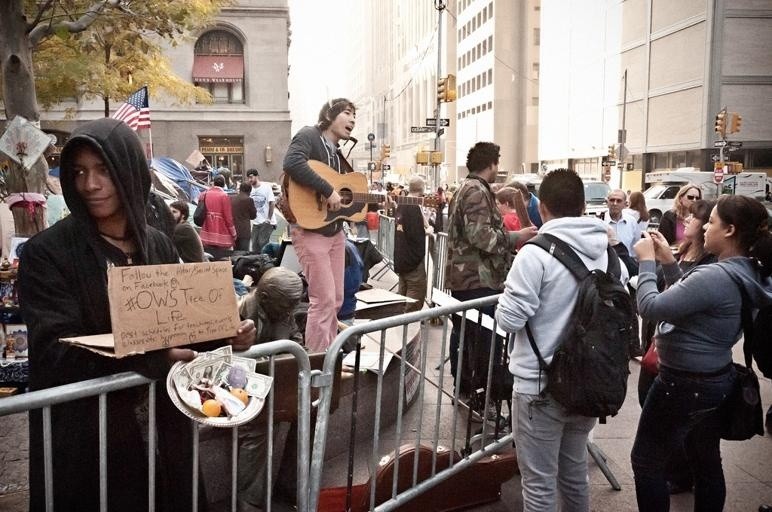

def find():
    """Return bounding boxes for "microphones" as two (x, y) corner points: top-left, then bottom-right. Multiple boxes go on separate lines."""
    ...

(349, 136), (359, 144)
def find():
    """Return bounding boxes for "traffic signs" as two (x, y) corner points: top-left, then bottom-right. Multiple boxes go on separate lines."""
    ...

(438, 128), (444, 137)
(425, 117), (450, 128)
(601, 160), (616, 166)
(713, 139), (743, 152)
(710, 153), (730, 162)
(411, 126), (435, 132)
(383, 164), (391, 170)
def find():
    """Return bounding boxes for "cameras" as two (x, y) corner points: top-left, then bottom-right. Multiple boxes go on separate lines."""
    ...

(641, 232), (653, 238)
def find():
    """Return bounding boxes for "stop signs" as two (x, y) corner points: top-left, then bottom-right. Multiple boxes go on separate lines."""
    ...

(713, 167), (723, 184)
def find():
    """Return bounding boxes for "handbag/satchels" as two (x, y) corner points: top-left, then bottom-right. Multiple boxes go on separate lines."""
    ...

(193, 191), (210, 228)
(718, 360), (765, 443)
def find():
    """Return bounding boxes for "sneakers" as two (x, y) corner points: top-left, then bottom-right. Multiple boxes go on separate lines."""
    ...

(469, 403), (512, 423)
(448, 390), (480, 407)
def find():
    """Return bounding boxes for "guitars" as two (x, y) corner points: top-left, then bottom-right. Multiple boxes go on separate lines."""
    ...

(280, 159), (441, 230)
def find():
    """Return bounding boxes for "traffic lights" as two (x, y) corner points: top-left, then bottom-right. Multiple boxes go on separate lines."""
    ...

(437, 77), (450, 102)
(713, 111), (726, 134)
(608, 144), (615, 158)
(731, 112), (742, 134)
(382, 143), (391, 158)
(368, 162), (376, 171)
(626, 162), (634, 171)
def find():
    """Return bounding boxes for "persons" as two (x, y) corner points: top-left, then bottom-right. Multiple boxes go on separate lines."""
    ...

(20, 100), (771, 512)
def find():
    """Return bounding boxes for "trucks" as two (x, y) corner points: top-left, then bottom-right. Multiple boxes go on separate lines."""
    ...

(640, 169), (768, 230)
(583, 177), (616, 222)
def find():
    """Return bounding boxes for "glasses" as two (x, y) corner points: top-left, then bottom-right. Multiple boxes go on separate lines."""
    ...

(684, 194), (700, 201)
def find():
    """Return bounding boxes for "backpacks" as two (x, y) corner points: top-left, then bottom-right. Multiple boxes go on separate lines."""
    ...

(518, 234), (633, 425)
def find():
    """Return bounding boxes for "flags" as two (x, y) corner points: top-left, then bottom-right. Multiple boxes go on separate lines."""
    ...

(113, 85), (152, 128)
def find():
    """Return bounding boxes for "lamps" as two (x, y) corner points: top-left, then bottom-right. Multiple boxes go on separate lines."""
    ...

(265, 144), (272, 163)
